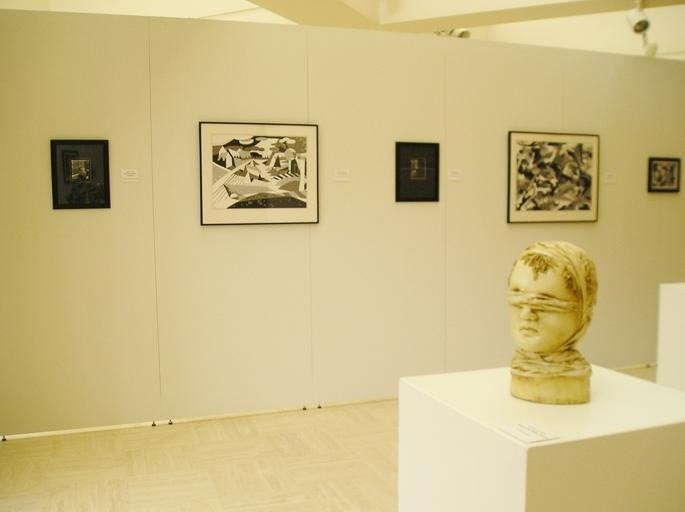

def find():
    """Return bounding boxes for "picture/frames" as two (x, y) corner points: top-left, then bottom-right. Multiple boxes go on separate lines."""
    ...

(395, 142), (439, 202)
(199, 121), (319, 225)
(507, 130), (599, 223)
(648, 157), (680, 192)
(50, 139), (111, 209)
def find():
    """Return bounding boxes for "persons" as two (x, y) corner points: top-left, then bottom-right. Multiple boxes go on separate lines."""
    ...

(505, 237), (600, 378)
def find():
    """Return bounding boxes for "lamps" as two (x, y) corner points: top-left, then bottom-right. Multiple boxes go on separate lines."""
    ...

(626, 8), (659, 60)
(433, 26), (471, 39)
